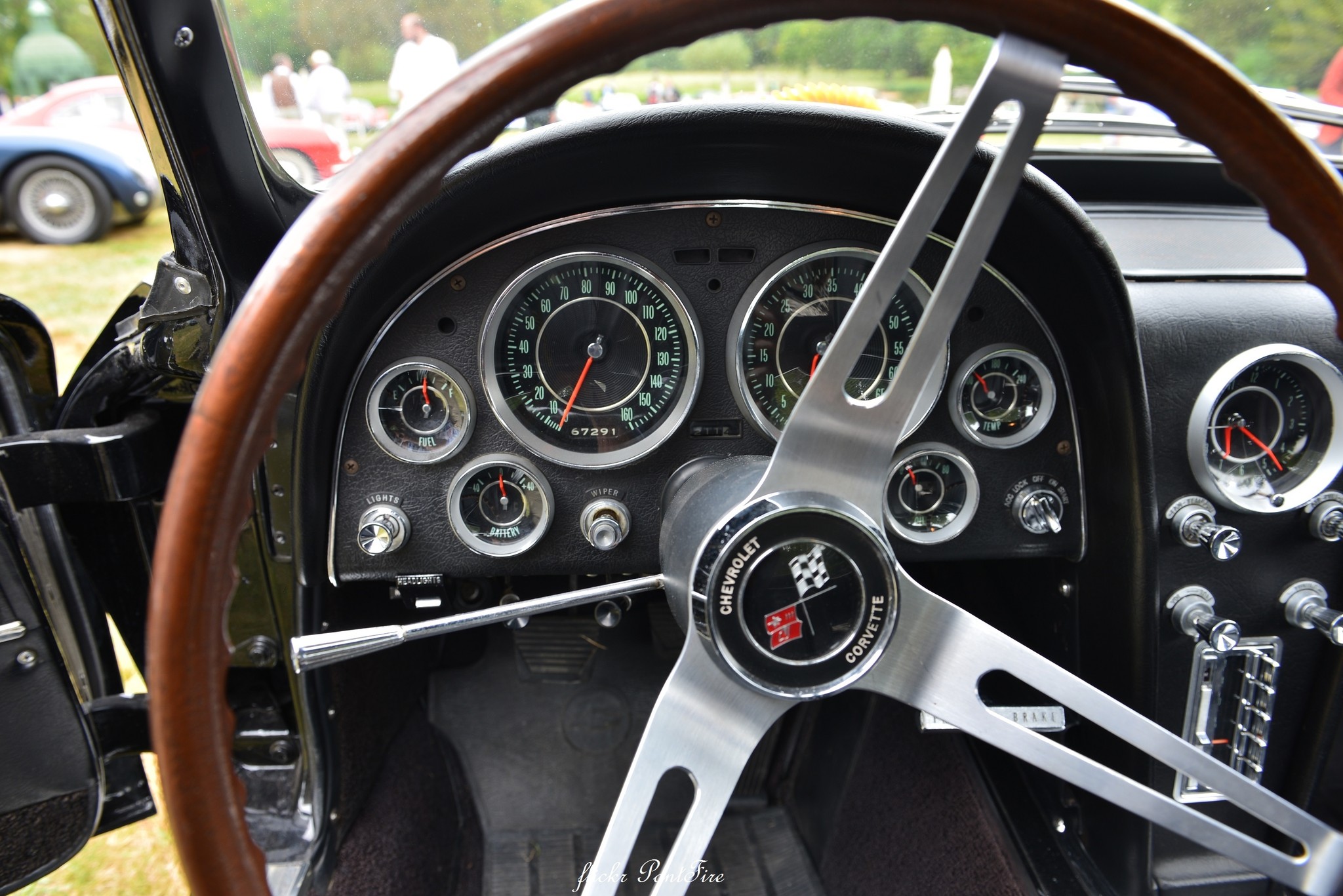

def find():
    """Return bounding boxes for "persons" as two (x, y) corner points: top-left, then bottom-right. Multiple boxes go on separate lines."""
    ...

(301, 50), (351, 127)
(386, 14), (459, 107)
(260, 52), (305, 122)
(526, 86), (680, 130)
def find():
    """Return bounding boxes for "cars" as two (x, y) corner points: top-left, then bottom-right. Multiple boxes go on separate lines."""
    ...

(0, 75), (343, 247)
